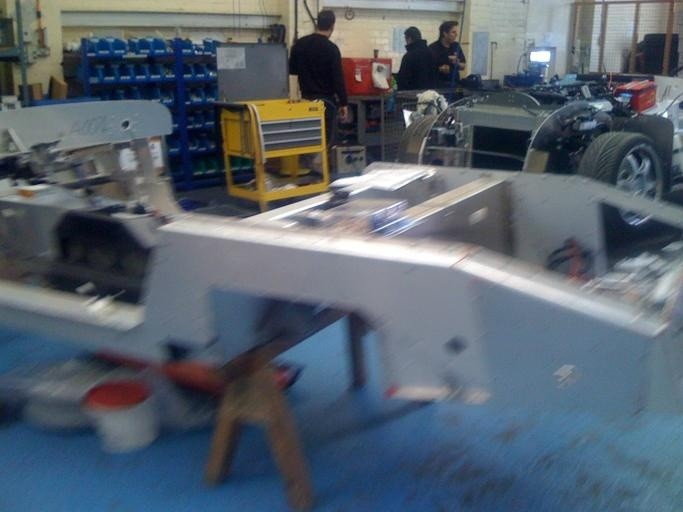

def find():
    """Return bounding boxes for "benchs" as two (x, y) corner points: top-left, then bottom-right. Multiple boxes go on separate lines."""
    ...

(205, 306), (365, 512)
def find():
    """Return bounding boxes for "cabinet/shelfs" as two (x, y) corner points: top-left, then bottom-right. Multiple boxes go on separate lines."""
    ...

(80, 37), (255, 191)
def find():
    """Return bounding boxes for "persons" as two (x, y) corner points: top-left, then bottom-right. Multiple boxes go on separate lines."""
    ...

(430, 21), (467, 88)
(395, 26), (432, 90)
(288, 10), (348, 144)
